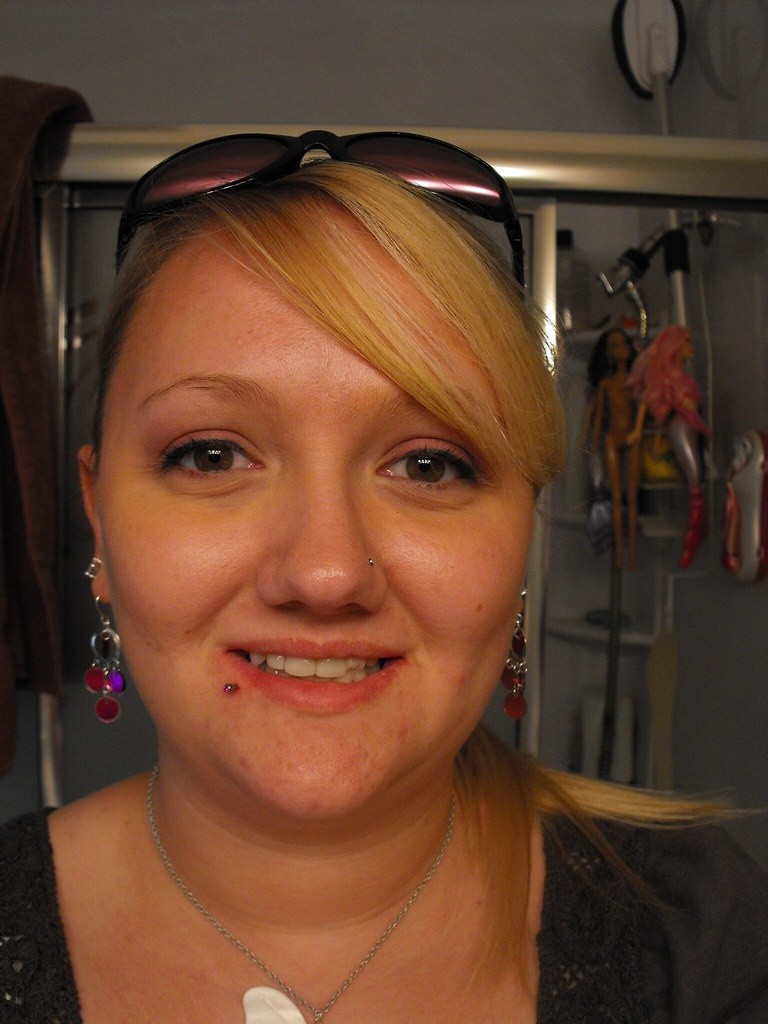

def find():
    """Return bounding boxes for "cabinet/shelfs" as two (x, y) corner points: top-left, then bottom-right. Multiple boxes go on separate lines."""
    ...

(527, 331), (672, 790)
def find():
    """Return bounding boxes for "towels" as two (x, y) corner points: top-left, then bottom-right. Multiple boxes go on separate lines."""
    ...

(0, 75), (91, 742)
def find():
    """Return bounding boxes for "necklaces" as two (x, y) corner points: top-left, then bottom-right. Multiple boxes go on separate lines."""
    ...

(145, 768), (457, 1023)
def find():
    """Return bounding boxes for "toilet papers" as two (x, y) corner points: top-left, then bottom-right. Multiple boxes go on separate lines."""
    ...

(579, 693), (635, 784)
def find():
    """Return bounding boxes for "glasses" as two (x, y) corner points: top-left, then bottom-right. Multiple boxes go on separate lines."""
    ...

(114, 130), (524, 302)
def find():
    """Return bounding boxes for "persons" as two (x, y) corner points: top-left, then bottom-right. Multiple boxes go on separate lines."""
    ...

(0, 160), (768, 1024)
